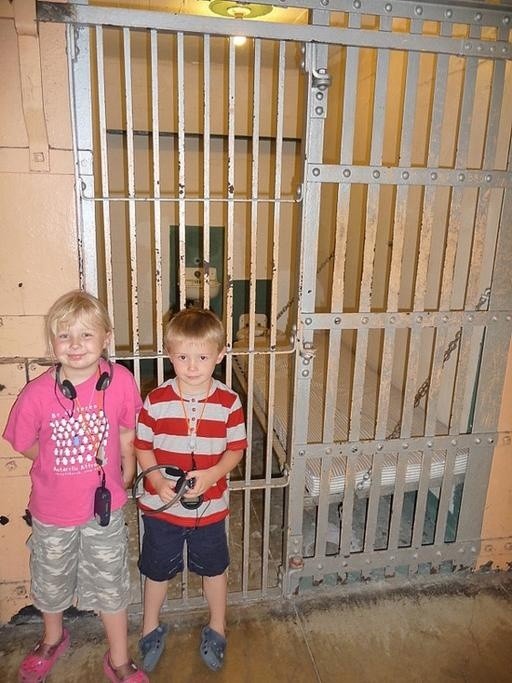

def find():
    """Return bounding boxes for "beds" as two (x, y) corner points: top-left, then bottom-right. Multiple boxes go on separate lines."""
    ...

(230, 327), (469, 550)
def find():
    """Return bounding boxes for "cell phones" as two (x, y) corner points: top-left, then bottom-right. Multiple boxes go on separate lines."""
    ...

(93, 486), (110, 527)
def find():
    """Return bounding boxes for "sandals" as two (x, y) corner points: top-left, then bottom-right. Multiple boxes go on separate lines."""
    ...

(102, 647), (149, 683)
(139, 622), (169, 671)
(19, 629), (70, 683)
(201, 626), (227, 671)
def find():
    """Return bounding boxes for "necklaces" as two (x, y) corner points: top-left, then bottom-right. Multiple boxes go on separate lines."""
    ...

(177, 376), (213, 434)
(60, 361), (103, 451)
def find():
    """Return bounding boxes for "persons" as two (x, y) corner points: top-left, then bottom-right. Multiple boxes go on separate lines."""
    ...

(1, 286), (150, 683)
(132, 306), (249, 676)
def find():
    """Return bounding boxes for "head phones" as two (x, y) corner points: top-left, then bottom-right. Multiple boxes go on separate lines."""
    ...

(132, 464), (204, 514)
(56, 353), (113, 400)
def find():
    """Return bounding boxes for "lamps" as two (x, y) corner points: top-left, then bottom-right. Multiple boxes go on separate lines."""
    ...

(226, 6), (251, 47)
